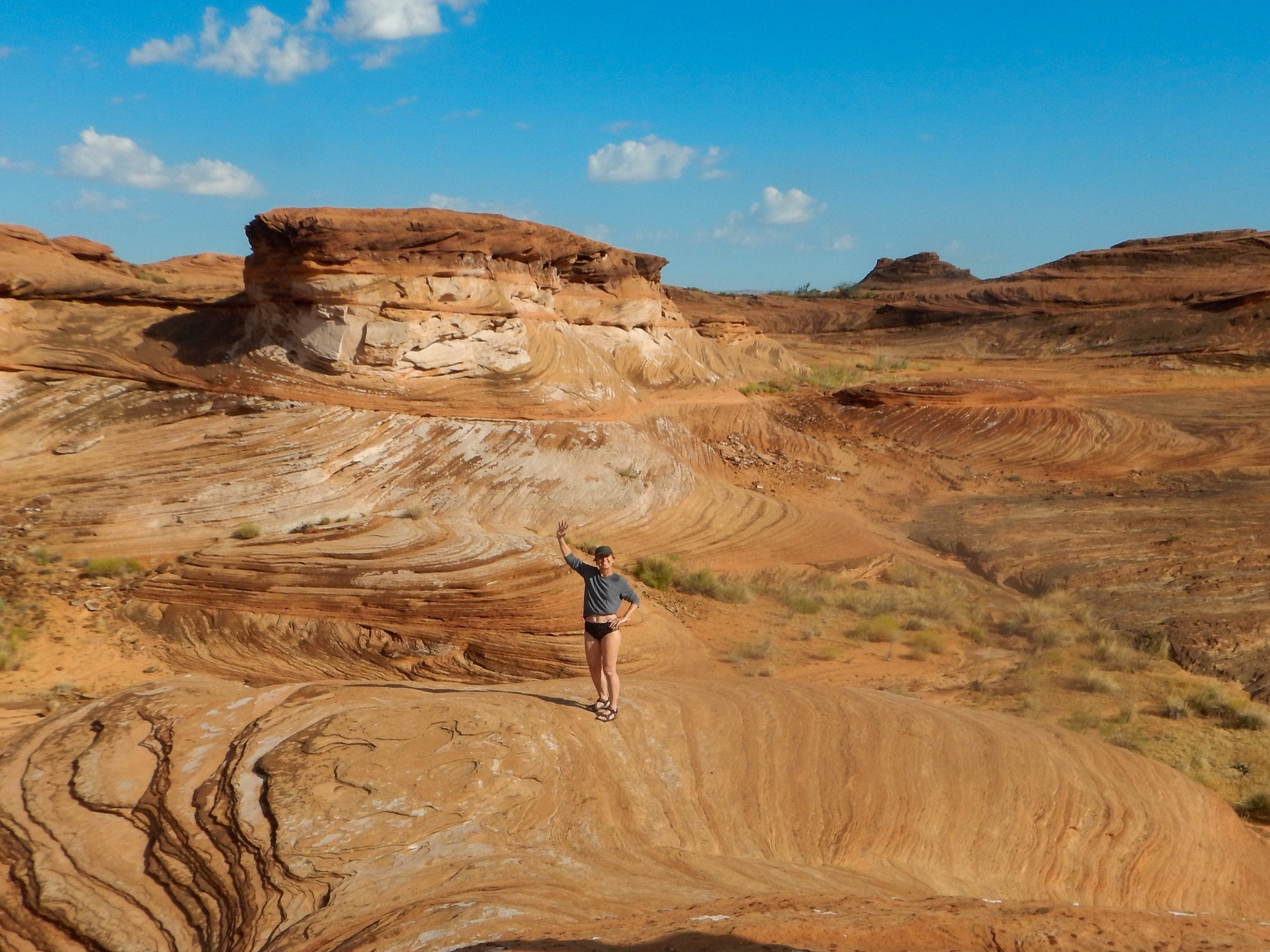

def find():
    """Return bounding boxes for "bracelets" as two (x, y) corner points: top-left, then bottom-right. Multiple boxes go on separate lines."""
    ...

(622, 615), (628, 622)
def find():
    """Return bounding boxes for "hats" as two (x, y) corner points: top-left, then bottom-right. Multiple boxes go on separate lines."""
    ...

(594, 545), (613, 556)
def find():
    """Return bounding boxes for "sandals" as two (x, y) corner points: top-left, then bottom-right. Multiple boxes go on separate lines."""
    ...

(586, 697), (610, 712)
(596, 707), (618, 721)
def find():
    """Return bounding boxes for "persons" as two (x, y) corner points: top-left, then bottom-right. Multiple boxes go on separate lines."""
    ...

(557, 520), (640, 722)
